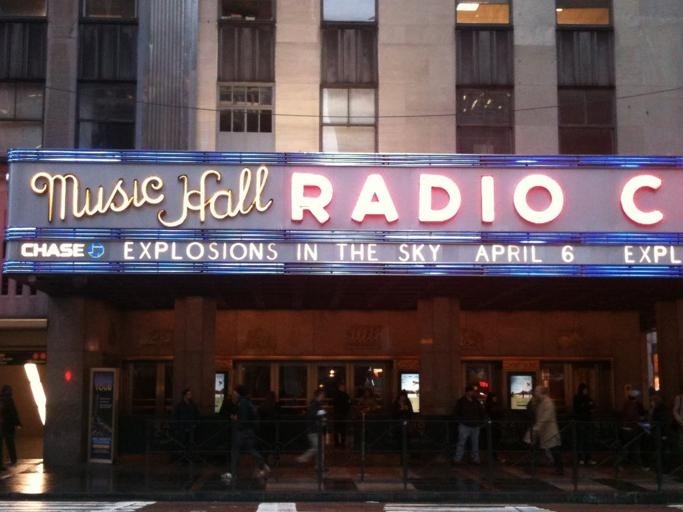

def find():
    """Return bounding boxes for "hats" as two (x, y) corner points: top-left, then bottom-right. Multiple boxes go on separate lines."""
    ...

(465, 385), (473, 392)
(233, 383), (250, 396)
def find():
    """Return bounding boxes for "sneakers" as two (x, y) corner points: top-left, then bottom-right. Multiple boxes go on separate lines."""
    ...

(223, 469), (236, 481)
(253, 468), (267, 479)
(579, 458), (598, 467)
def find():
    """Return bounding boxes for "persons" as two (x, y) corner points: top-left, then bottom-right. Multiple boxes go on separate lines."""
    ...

(387, 388), (418, 465)
(453, 386), (486, 468)
(172, 388), (199, 465)
(619, 387), (682, 484)
(293, 389), (329, 471)
(0, 383), (24, 468)
(357, 387), (376, 405)
(218, 385), (270, 482)
(527, 384), (560, 463)
(326, 379), (353, 451)
(571, 382), (598, 464)
(257, 391), (281, 465)
